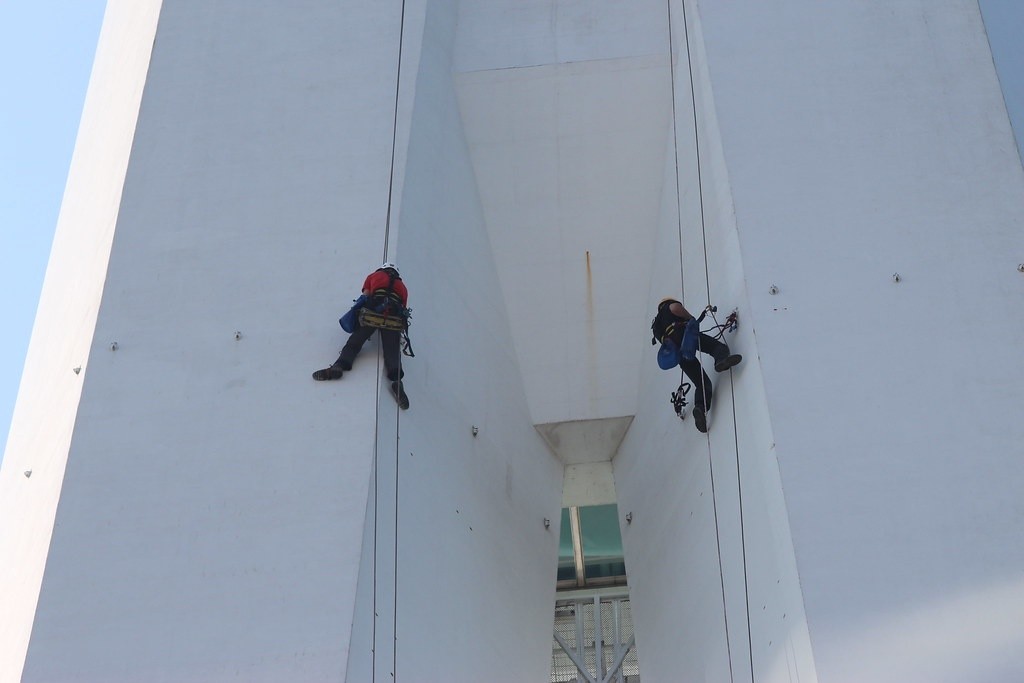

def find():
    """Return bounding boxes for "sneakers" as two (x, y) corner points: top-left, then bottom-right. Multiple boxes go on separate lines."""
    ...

(715, 354), (742, 372)
(693, 406), (707, 433)
(392, 381), (409, 410)
(312, 367), (342, 381)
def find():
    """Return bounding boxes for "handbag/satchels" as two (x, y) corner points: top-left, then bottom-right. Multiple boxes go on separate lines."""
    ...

(339, 300), (366, 333)
(657, 337), (680, 370)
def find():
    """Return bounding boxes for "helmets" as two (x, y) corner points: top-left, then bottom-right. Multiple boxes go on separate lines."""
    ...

(381, 262), (400, 275)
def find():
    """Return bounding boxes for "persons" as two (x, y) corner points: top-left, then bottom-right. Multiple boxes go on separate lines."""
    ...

(650, 297), (743, 433)
(311, 262), (410, 410)
